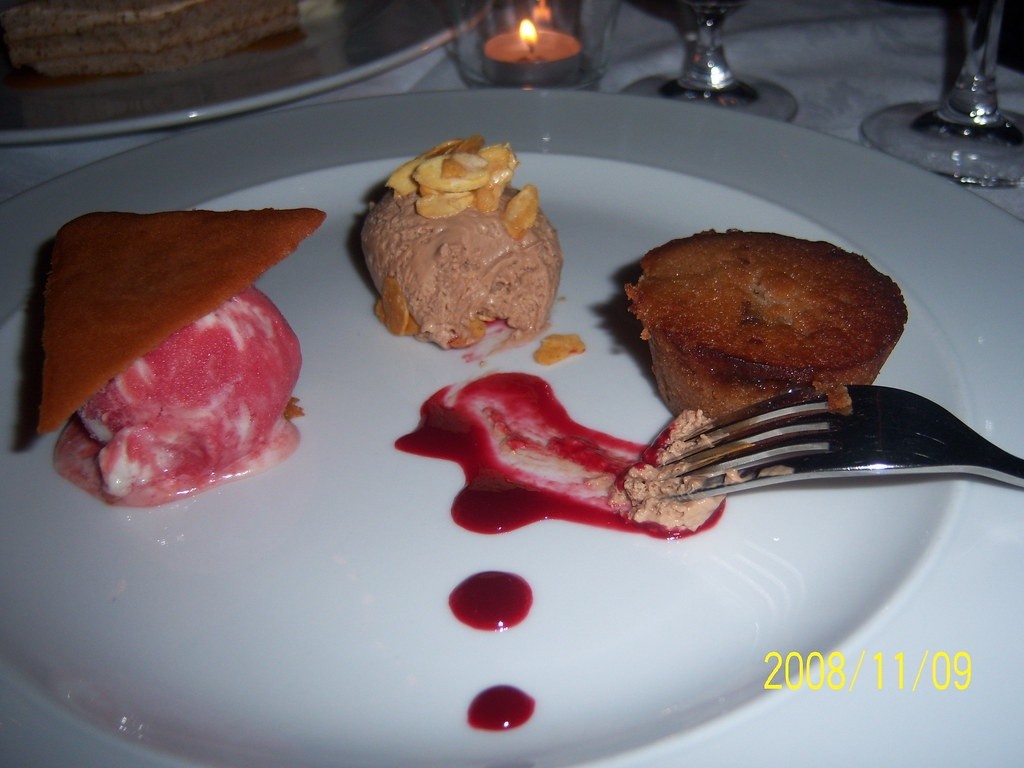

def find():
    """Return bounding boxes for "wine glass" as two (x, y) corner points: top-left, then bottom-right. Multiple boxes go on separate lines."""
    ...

(616, 0), (798, 124)
(858, 1), (1024, 188)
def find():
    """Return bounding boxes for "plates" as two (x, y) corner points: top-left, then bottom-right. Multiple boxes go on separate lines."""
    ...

(0, 0), (492, 145)
(0, 154), (966, 767)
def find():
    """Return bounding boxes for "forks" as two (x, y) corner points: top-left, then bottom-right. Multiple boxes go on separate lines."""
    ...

(663, 385), (1024, 505)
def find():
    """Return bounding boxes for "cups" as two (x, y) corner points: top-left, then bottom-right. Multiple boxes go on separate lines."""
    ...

(451, 0), (622, 90)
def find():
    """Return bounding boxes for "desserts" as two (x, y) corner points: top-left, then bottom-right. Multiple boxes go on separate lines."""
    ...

(38, 206), (327, 500)
(360, 134), (560, 351)
(615, 414), (731, 531)
